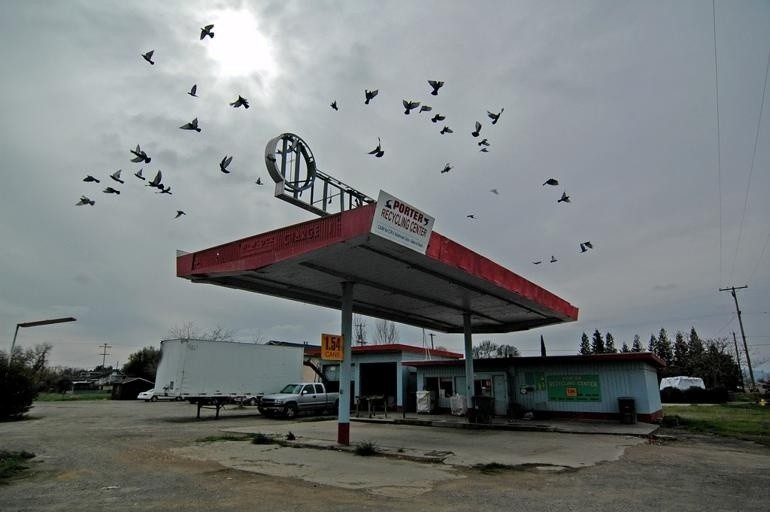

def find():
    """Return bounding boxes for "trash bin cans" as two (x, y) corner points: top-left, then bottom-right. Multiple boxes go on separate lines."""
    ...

(617, 396), (635, 425)
(471, 393), (494, 422)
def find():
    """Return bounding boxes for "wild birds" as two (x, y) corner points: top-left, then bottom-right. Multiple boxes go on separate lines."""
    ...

(400, 81), (506, 153)
(330, 89), (379, 111)
(141, 50), (154, 65)
(490, 188), (499, 195)
(543, 178), (572, 204)
(230, 95), (250, 109)
(75, 142), (186, 219)
(466, 214), (475, 219)
(580, 241), (594, 254)
(368, 137), (384, 158)
(532, 255), (558, 265)
(218, 155), (233, 174)
(179, 84), (201, 133)
(199, 24), (215, 40)
(256, 177), (263, 186)
(439, 164), (451, 174)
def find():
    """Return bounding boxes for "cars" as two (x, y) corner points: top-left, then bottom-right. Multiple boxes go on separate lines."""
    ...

(136, 388), (185, 402)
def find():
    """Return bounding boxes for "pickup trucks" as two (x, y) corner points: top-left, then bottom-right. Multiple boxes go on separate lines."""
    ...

(257, 382), (339, 421)
